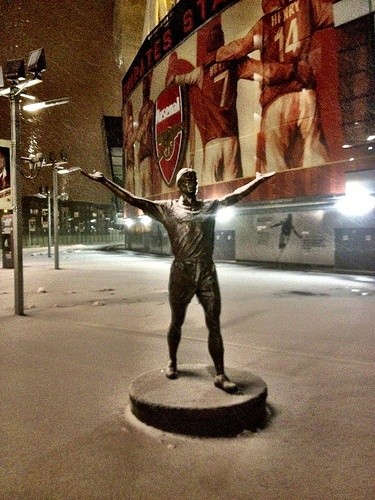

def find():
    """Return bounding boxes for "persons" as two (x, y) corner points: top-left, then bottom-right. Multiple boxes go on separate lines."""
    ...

(167, 23), (313, 187)
(77, 167), (278, 394)
(123, 100), (154, 195)
(201, 0), (337, 173)
(135, 74), (155, 195)
(165, 51), (196, 169)
(271, 213), (302, 250)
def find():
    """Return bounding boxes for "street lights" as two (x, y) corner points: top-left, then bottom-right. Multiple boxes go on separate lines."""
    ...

(0, 48), (71, 316)
(20, 134), (80, 269)
(34, 185), (69, 258)
